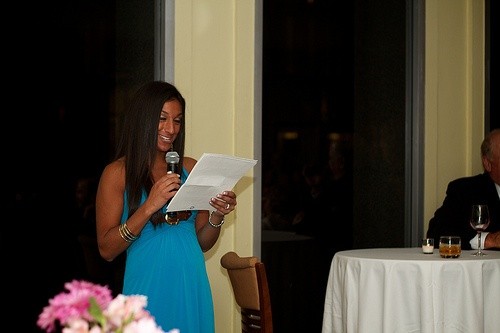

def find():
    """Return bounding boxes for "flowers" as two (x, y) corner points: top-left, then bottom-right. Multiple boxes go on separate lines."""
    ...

(36, 281), (181, 333)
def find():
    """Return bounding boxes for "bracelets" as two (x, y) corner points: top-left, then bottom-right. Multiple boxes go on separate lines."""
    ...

(209, 214), (224, 227)
(119, 222), (136, 242)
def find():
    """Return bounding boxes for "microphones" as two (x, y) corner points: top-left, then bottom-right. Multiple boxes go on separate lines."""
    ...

(165, 152), (180, 225)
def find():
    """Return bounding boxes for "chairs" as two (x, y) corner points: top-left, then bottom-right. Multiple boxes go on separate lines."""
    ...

(220, 252), (273, 333)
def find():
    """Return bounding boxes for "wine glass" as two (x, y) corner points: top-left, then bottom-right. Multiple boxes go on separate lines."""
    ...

(470, 205), (490, 256)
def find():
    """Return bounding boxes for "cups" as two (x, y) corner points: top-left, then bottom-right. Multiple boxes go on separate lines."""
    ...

(422, 238), (434, 254)
(438, 236), (461, 258)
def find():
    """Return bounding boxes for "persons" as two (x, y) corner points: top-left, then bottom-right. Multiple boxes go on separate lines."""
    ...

(426, 125), (500, 249)
(97, 81), (236, 333)
(264, 141), (354, 249)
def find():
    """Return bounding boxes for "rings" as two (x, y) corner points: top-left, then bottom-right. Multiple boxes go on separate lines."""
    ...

(225, 204), (229, 209)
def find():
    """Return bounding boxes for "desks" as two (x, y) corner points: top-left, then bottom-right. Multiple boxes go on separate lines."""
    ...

(321, 248), (500, 333)
